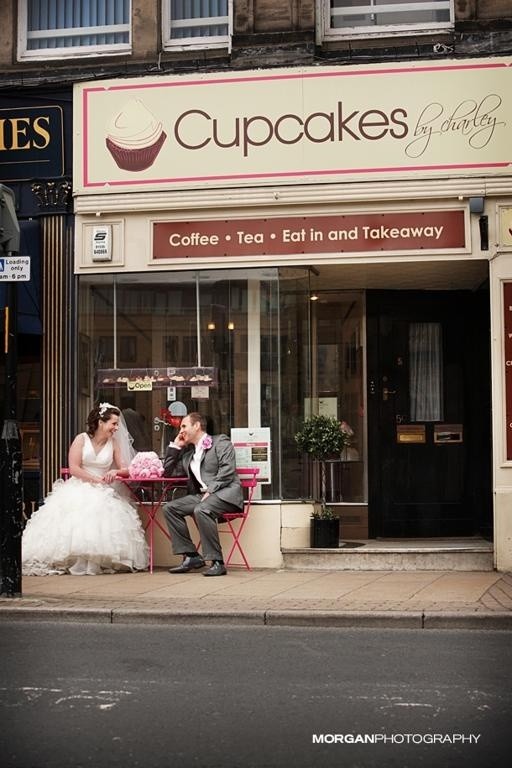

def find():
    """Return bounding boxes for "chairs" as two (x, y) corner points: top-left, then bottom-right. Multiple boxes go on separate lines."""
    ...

(59, 466), (257, 573)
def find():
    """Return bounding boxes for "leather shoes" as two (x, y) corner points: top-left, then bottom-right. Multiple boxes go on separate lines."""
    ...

(204, 561), (227, 575)
(169, 554), (204, 573)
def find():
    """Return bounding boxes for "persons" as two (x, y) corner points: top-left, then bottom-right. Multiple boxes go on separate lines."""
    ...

(163, 412), (243, 575)
(23, 401), (147, 575)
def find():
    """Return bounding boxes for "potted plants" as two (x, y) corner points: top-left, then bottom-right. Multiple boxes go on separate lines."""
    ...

(294, 414), (353, 548)
